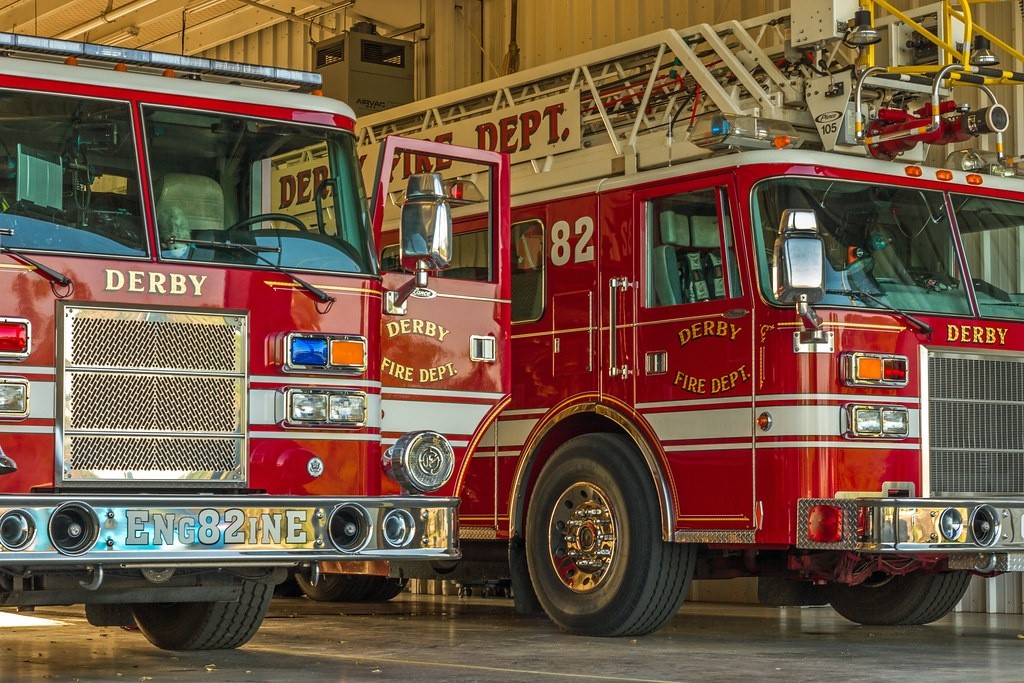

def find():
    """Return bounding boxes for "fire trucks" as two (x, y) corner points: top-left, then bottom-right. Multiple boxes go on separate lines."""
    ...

(289, 57), (1024, 636)
(0, 28), (512, 650)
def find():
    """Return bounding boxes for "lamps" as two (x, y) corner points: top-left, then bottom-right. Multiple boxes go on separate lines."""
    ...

(969, 34), (1000, 65)
(845, 11), (882, 80)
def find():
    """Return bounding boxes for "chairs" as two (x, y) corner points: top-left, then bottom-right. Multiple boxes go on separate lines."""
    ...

(153, 172), (224, 256)
(653, 210), (914, 314)
(59, 190), (140, 238)
